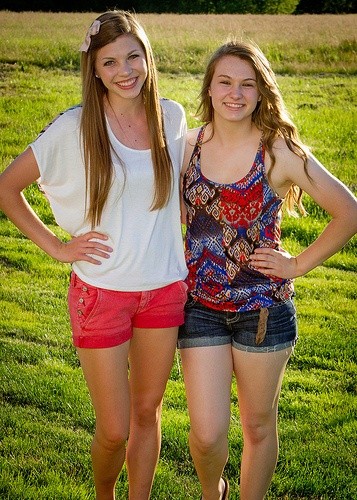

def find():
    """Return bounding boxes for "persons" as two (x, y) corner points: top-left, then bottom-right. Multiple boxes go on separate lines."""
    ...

(175, 37), (356, 500)
(0, 13), (192, 498)
